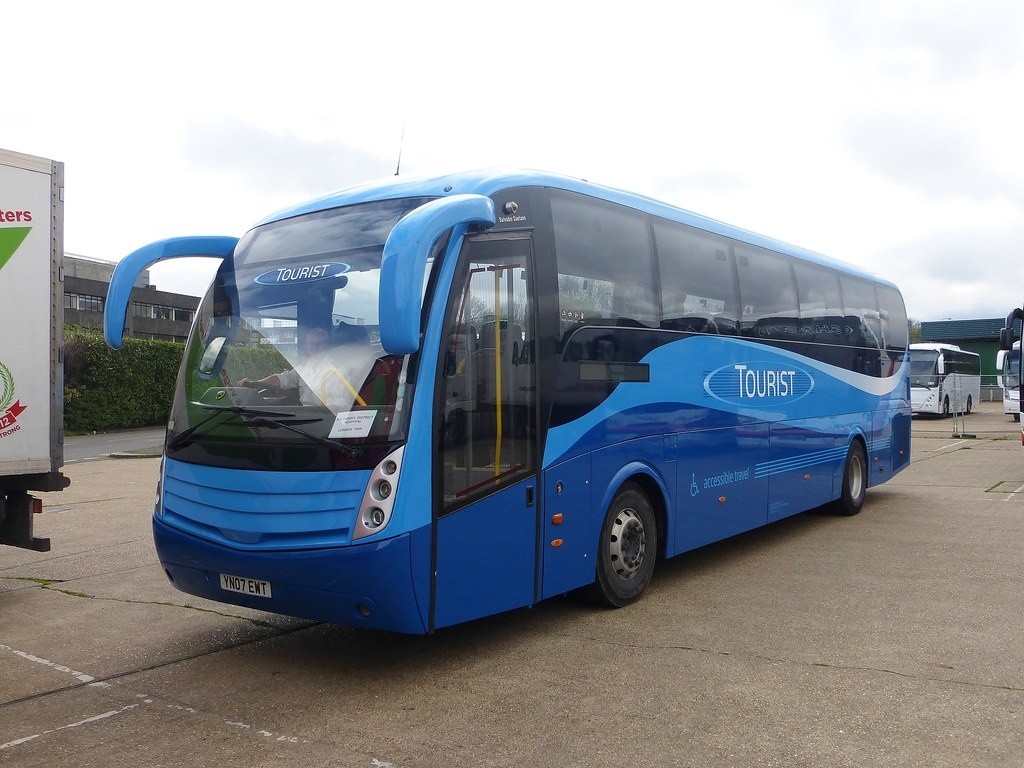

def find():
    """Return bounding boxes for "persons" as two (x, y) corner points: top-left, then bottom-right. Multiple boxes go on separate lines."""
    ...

(236, 329), (350, 408)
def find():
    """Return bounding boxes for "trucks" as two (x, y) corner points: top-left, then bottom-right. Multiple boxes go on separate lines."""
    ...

(0, 143), (79, 556)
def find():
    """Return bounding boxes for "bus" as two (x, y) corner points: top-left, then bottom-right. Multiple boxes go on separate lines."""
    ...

(100, 168), (916, 637)
(907, 343), (982, 418)
(995, 304), (1024, 450)
